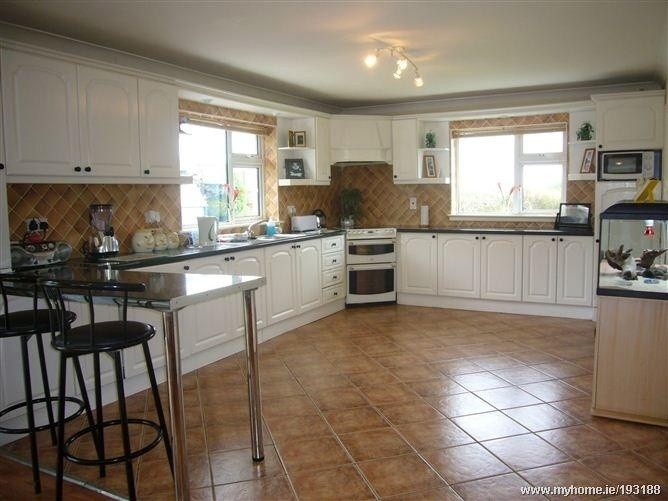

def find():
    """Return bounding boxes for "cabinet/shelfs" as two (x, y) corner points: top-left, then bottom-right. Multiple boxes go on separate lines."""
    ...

(276, 114), (450, 187)
(0, 47), (140, 179)
(438, 233), (594, 307)
(321, 235), (346, 306)
(566, 109), (599, 182)
(137, 77), (181, 179)
(592, 93), (664, 308)
(395, 232), (437, 297)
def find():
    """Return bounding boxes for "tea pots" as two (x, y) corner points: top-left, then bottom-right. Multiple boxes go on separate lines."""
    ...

(316, 214), (327, 229)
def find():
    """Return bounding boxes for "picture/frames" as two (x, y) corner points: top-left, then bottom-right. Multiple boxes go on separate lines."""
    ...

(580, 148), (596, 175)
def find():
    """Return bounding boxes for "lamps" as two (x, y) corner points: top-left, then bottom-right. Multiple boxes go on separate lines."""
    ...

(363, 46), (425, 87)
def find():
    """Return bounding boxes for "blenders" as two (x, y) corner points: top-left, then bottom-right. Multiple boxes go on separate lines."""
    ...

(89, 203), (119, 256)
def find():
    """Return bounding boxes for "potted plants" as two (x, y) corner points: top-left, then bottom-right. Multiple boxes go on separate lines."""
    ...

(575, 121), (595, 141)
(338, 188), (363, 227)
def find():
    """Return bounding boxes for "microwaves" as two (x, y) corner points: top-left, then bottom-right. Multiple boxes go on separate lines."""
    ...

(600, 152), (655, 180)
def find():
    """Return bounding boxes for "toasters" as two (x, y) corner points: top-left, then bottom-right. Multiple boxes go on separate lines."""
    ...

(291, 214), (318, 232)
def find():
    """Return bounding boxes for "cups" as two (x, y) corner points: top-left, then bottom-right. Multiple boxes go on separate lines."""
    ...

(266, 217), (274, 235)
(197, 216), (219, 244)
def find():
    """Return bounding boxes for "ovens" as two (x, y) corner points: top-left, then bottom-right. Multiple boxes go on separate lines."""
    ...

(347, 229), (397, 305)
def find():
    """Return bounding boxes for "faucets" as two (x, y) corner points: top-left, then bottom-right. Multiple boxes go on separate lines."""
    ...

(245, 218), (269, 238)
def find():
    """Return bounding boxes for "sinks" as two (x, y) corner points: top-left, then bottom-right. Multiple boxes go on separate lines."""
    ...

(217, 233), (248, 242)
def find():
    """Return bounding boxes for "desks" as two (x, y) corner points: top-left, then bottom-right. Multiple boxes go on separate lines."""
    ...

(1, 265), (267, 501)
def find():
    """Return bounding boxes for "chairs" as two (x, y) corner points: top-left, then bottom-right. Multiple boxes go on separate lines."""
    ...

(0, 273), (175, 501)
